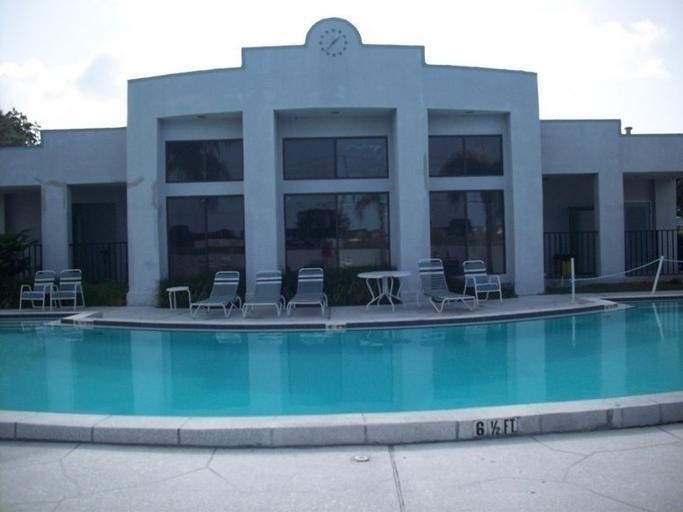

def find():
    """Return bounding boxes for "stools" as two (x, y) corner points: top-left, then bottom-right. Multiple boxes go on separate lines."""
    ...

(165, 286), (191, 313)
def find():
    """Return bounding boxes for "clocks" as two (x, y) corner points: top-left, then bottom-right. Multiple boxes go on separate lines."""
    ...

(316, 27), (349, 59)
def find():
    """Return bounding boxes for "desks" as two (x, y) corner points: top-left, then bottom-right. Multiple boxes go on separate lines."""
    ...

(356, 270), (411, 311)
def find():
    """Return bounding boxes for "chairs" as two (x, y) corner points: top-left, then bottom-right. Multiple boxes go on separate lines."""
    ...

(191, 267), (329, 318)
(18, 269), (87, 311)
(416, 257), (503, 313)
